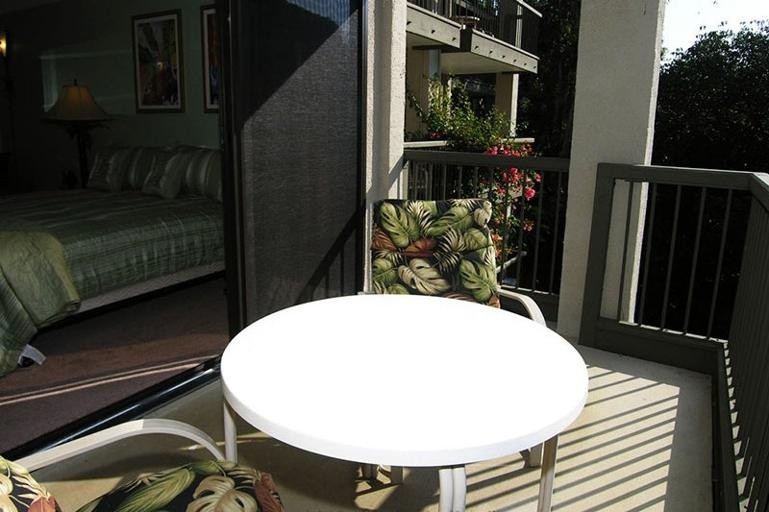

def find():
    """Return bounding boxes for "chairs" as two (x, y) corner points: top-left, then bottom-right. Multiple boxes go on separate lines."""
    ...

(0, 416), (286, 512)
(357, 194), (546, 478)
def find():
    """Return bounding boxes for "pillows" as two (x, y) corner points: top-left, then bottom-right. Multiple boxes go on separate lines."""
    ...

(83, 144), (223, 201)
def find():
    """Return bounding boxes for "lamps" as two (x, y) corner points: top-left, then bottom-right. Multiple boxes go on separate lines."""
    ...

(38, 77), (115, 188)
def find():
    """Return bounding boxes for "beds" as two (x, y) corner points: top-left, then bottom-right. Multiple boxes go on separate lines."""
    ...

(0, 185), (225, 368)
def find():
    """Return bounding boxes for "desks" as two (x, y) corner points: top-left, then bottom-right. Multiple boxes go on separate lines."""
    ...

(219, 290), (590, 512)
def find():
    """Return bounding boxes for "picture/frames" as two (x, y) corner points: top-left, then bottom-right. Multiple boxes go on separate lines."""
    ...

(129, 7), (186, 115)
(200, 3), (222, 113)
